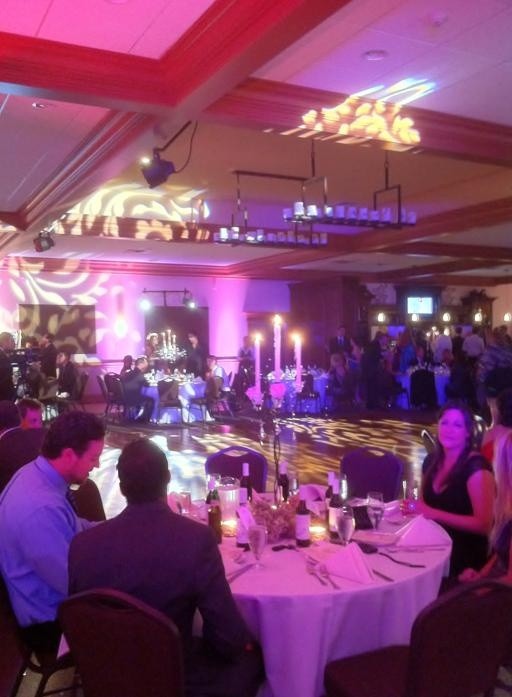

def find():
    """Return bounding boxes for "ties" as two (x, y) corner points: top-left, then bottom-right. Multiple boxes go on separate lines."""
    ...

(67, 487), (80, 517)
(339, 336), (344, 346)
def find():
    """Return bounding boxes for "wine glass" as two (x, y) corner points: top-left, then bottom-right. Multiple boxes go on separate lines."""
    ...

(333, 505), (357, 553)
(246, 523), (270, 573)
(366, 490), (385, 540)
(206, 473), (222, 500)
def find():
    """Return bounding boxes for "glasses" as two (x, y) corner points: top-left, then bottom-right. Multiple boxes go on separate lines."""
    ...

(438, 417), (465, 431)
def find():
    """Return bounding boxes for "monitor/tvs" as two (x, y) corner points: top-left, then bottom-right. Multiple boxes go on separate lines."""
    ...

(407, 297), (433, 314)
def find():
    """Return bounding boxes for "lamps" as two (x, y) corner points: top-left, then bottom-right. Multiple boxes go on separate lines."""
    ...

(502, 312), (510, 322)
(190, 296), (196, 312)
(439, 312), (450, 322)
(142, 293), (151, 311)
(115, 295), (126, 337)
(377, 308), (387, 323)
(473, 311), (482, 323)
(411, 313), (418, 324)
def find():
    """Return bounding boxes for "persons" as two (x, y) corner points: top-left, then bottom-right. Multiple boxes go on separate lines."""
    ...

(400, 402), (495, 595)
(40, 333), (58, 379)
(145, 335), (160, 371)
(237, 336), (255, 387)
(206, 355), (230, 399)
(184, 329), (208, 380)
(0, 409), (103, 641)
(67, 436), (266, 696)
(358, 329), (419, 409)
(118, 356), (154, 424)
(451, 328), (465, 355)
(349, 336), (365, 410)
(54, 351), (81, 400)
(20, 337), (45, 399)
(120, 355), (132, 374)
(412, 347), (429, 369)
(492, 394), (512, 556)
(328, 326), (350, 355)
(440, 348), (455, 368)
(462, 327), (486, 365)
(431, 326), (452, 363)
(18, 398), (43, 429)
(475, 329), (508, 396)
(0, 332), (17, 401)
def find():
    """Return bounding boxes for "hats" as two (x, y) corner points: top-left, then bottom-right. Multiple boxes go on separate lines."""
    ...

(485, 369), (510, 398)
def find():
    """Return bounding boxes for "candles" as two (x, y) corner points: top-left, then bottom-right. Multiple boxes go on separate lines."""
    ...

(295, 334), (303, 394)
(253, 334), (260, 410)
(161, 329), (177, 350)
(271, 314), (282, 384)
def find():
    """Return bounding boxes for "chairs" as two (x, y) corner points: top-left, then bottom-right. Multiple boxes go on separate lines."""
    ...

(56, 590), (185, 697)
(340, 447), (410, 502)
(98, 373), (128, 423)
(188, 377), (224, 423)
(204, 448), (266, 497)
(322, 581), (511, 697)
(156, 379), (186, 425)
(409, 369), (437, 412)
(0, 586), (77, 697)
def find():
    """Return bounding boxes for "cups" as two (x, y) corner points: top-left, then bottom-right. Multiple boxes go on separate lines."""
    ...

(308, 501), (330, 546)
(217, 477), (239, 540)
(402, 480), (419, 520)
(274, 480), (286, 512)
(175, 491), (192, 515)
(327, 473), (353, 512)
(288, 477), (300, 498)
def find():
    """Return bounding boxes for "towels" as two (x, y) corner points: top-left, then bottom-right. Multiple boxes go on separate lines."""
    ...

(297, 482), (330, 505)
(383, 501), (403, 524)
(314, 540), (377, 584)
(394, 513), (453, 546)
(217, 542), (256, 582)
(167, 493), (181, 514)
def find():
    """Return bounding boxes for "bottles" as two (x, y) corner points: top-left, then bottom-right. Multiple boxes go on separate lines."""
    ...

(296, 484), (311, 549)
(327, 479), (343, 541)
(239, 462), (253, 510)
(275, 458), (291, 505)
(207, 477), (222, 546)
(324, 469), (337, 523)
